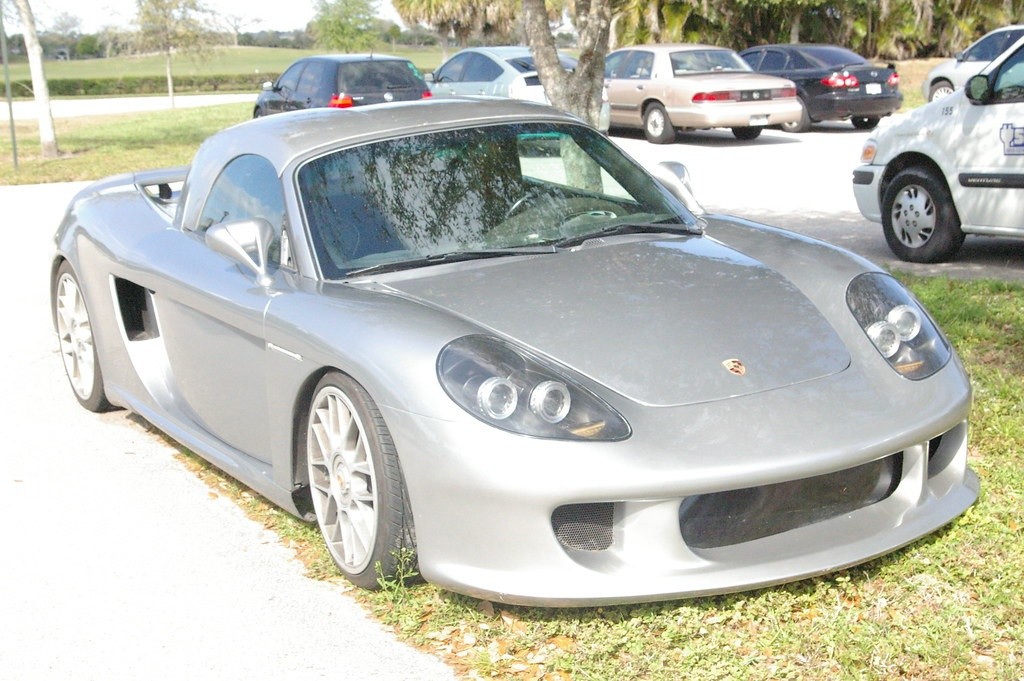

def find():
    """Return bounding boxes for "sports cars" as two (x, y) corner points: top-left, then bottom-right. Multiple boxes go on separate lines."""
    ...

(47, 94), (987, 611)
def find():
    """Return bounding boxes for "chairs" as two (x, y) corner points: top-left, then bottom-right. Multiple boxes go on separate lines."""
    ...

(315, 192), (404, 262)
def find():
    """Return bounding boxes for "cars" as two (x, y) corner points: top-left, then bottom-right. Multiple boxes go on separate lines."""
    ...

(249, 52), (434, 122)
(601, 44), (804, 146)
(924, 22), (1024, 103)
(849, 33), (1023, 263)
(737, 42), (905, 134)
(425, 46), (611, 154)
